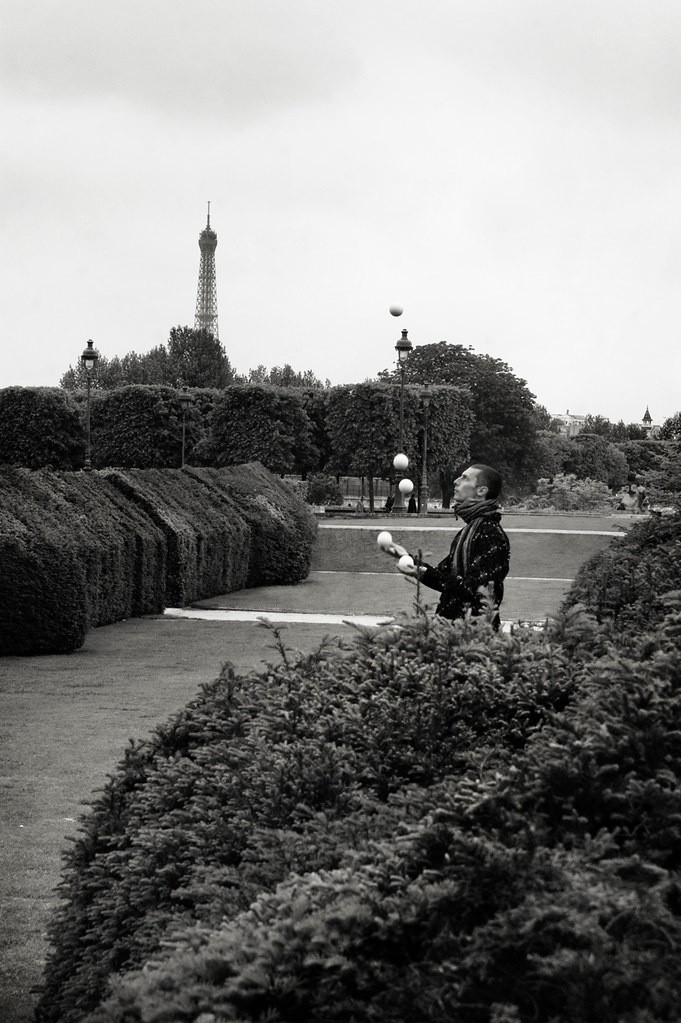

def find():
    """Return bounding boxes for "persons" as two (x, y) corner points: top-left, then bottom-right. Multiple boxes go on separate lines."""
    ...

(381, 465), (510, 633)
(356, 496), (365, 513)
(384, 492), (417, 513)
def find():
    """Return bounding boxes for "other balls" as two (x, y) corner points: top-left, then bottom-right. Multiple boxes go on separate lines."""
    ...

(399, 555), (414, 568)
(390, 303), (403, 317)
(377, 531), (392, 547)
(393, 453), (408, 469)
(399, 479), (414, 493)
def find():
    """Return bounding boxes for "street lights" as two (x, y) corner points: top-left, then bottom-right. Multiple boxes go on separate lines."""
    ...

(418, 382), (430, 516)
(79, 338), (100, 472)
(389, 328), (414, 517)
(178, 386), (189, 468)
